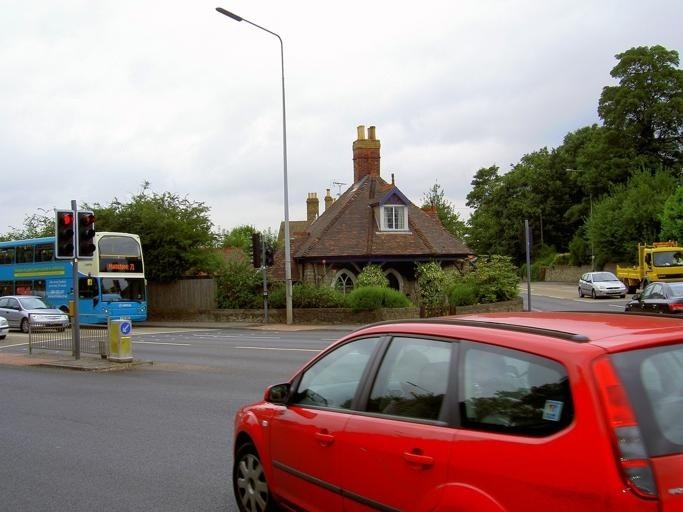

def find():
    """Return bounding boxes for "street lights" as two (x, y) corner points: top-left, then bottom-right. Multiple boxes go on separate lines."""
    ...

(215, 7), (293, 325)
(566, 168), (594, 270)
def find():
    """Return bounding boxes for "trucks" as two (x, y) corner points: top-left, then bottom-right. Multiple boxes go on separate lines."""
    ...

(616, 240), (683, 288)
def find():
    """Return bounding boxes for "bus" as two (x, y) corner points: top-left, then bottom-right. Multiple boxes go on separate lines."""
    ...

(0, 232), (148, 326)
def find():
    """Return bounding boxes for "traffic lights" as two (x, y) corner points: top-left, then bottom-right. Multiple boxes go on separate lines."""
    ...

(54, 209), (96, 260)
(252, 233), (273, 268)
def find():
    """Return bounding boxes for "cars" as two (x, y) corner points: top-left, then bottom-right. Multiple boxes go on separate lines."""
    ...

(0, 295), (69, 339)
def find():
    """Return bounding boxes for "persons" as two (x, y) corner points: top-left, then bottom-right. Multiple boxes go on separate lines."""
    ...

(380, 349), (430, 413)
(671, 255), (682, 264)
